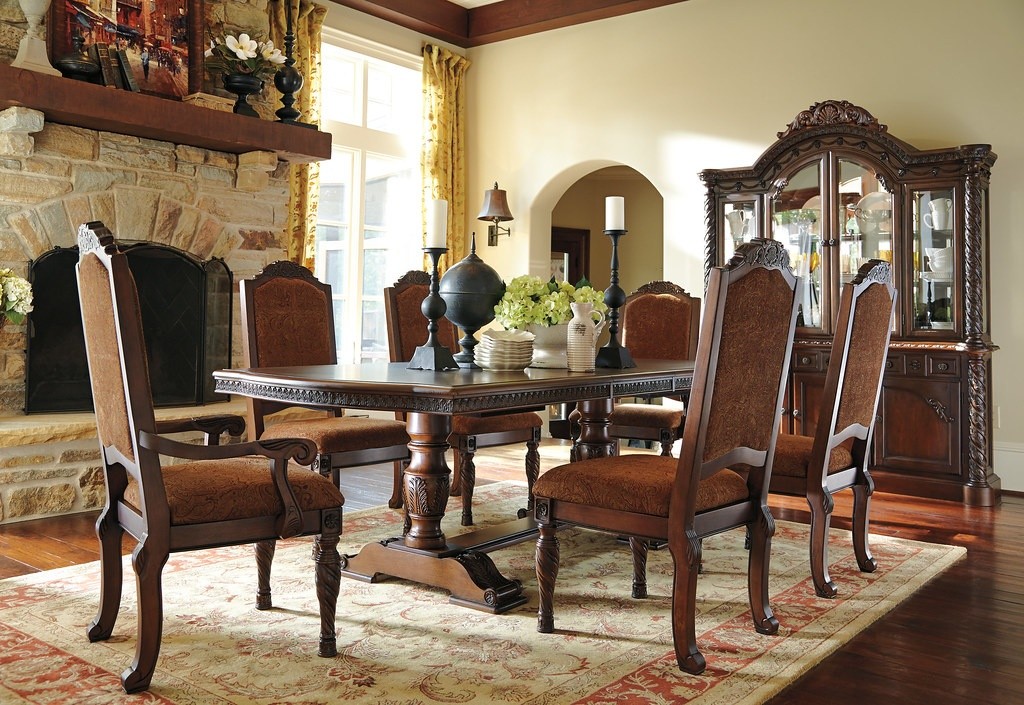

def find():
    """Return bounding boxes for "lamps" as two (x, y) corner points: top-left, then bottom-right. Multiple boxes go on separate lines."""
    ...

(476, 181), (514, 246)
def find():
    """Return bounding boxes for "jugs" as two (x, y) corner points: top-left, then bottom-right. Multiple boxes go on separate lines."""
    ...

(566, 302), (605, 373)
(726, 210), (745, 238)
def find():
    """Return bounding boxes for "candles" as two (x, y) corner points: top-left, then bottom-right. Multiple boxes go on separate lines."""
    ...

(605, 196), (625, 230)
(425, 199), (448, 248)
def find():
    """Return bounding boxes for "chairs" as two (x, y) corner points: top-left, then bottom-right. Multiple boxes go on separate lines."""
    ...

(607, 281), (701, 458)
(239, 259), (413, 537)
(530, 235), (803, 675)
(384, 270), (543, 526)
(698, 259), (898, 599)
(75, 220), (344, 694)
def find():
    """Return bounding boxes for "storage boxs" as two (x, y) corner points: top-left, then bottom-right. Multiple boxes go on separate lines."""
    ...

(181, 92), (235, 113)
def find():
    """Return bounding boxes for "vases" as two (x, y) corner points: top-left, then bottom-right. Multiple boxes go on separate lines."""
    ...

(225, 74), (262, 118)
(527, 324), (568, 368)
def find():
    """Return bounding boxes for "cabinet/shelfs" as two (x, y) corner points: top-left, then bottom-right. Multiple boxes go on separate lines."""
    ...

(697, 99), (999, 505)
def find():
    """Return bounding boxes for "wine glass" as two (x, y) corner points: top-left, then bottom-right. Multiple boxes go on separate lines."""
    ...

(774, 208), (819, 237)
(803, 253), (818, 282)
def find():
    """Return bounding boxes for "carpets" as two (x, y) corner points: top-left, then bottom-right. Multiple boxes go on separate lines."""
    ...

(0, 480), (969, 705)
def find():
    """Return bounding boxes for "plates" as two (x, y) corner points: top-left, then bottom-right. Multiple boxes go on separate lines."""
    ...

(931, 297), (951, 322)
(473, 328), (537, 373)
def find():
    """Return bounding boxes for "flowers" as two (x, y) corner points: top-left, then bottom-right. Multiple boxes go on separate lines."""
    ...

(0, 266), (35, 333)
(204, 21), (288, 101)
(494, 275), (611, 332)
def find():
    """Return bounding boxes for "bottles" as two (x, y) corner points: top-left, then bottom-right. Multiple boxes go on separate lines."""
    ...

(56, 36), (101, 83)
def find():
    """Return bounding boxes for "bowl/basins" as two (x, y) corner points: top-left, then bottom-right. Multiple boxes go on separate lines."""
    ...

(925, 246), (953, 272)
(855, 210), (891, 234)
(929, 321), (954, 330)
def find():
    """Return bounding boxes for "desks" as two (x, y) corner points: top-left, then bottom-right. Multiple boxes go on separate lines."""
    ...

(212, 356), (696, 615)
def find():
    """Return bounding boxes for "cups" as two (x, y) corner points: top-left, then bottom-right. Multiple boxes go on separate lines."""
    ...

(923, 210), (953, 230)
(841, 249), (892, 276)
(928, 198), (952, 211)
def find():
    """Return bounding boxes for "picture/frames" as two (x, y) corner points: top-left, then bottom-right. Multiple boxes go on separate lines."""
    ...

(46, 0), (204, 101)
